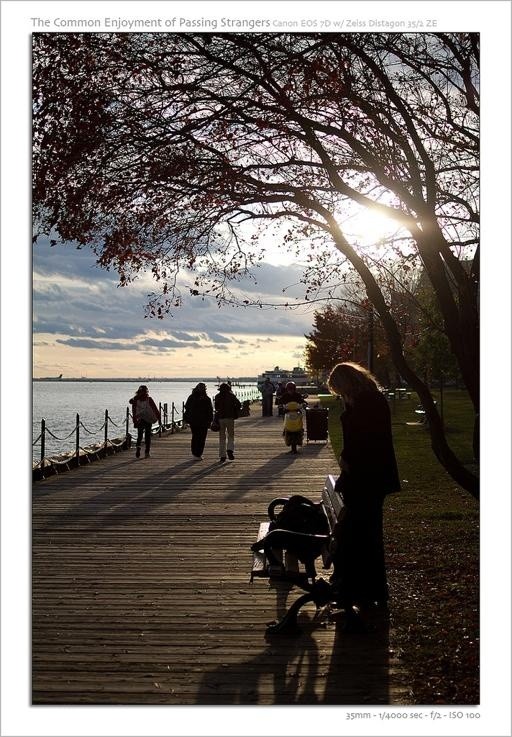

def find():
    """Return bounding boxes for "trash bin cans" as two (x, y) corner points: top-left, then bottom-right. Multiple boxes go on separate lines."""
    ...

(306, 407), (330, 445)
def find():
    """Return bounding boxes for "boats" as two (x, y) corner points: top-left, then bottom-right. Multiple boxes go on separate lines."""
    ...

(256, 359), (310, 388)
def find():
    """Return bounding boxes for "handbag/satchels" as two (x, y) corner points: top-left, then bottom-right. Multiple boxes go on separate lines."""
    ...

(212, 419), (219, 432)
(140, 406), (157, 423)
(269, 495), (326, 561)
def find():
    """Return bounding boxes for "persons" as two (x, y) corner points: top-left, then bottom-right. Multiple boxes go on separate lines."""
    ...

(211, 381), (242, 462)
(182, 382), (215, 461)
(326, 361), (402, 613)
(128, 384), (162, 459)
(278, 380), (309, 436)
(257, 377), (275, 417)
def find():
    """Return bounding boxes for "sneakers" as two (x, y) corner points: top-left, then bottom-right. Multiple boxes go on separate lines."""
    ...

(221, 450), (234, 461)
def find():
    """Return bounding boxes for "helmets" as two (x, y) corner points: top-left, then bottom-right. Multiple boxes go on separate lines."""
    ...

(286, 382), (296, 391)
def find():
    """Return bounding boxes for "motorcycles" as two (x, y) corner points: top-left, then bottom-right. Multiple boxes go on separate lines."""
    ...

(276, 393), (311, 453)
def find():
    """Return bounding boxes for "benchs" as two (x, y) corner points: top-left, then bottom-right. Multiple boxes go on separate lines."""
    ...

(250, 473), (345, 635)
